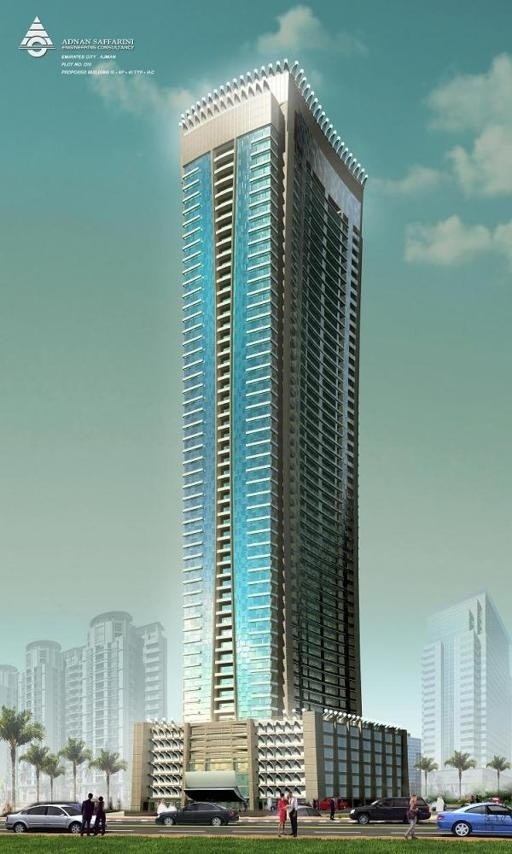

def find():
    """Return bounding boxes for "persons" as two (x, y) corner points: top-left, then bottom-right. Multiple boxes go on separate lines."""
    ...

(404, 795), (419, 838)
(157, 797), (177, 817)
(81, 793), (106, 837)
(330, 798), (335, 820)
(278, 791), (298, 837)
(470, 795), (480, 803)
